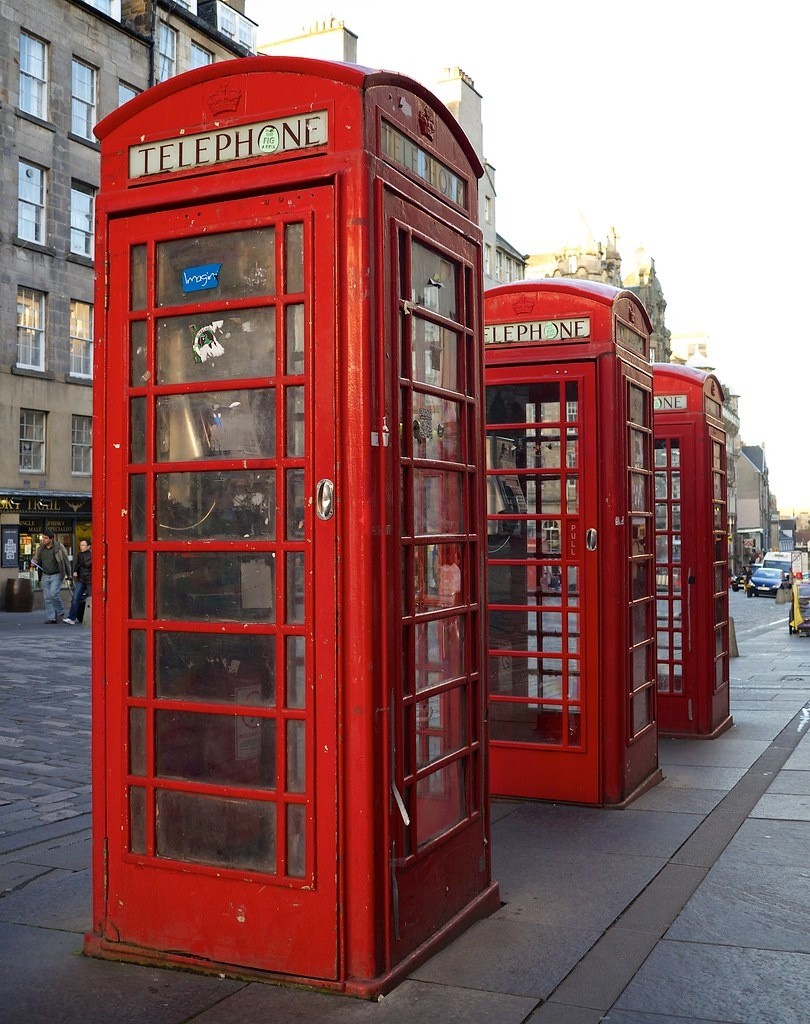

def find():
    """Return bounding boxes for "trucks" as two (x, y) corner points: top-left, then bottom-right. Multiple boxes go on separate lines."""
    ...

(761, 551), (792, 589)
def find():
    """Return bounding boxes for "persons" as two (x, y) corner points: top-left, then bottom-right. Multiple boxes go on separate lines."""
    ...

(30, 531), (72, 624)
(743, 565), (753, 593)
(62, 539), (92, 626)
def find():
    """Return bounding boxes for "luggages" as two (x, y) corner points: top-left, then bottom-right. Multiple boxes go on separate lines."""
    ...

(65, 578), (88, 623)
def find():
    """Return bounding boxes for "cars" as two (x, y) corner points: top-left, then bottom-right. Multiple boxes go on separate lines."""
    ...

(747, 568), (788, 598)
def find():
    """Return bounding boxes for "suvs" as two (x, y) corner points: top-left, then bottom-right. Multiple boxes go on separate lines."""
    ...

(731, 565), (761, 592)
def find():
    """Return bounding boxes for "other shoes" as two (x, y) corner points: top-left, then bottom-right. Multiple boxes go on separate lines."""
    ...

(55, 613), (64, 623)
(44, 619), (57, 624)
(62, 617), (75, 624)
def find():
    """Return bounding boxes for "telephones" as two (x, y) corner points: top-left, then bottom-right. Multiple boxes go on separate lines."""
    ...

(163, 332), (265, 507)
(484, 435), (528, 539)
